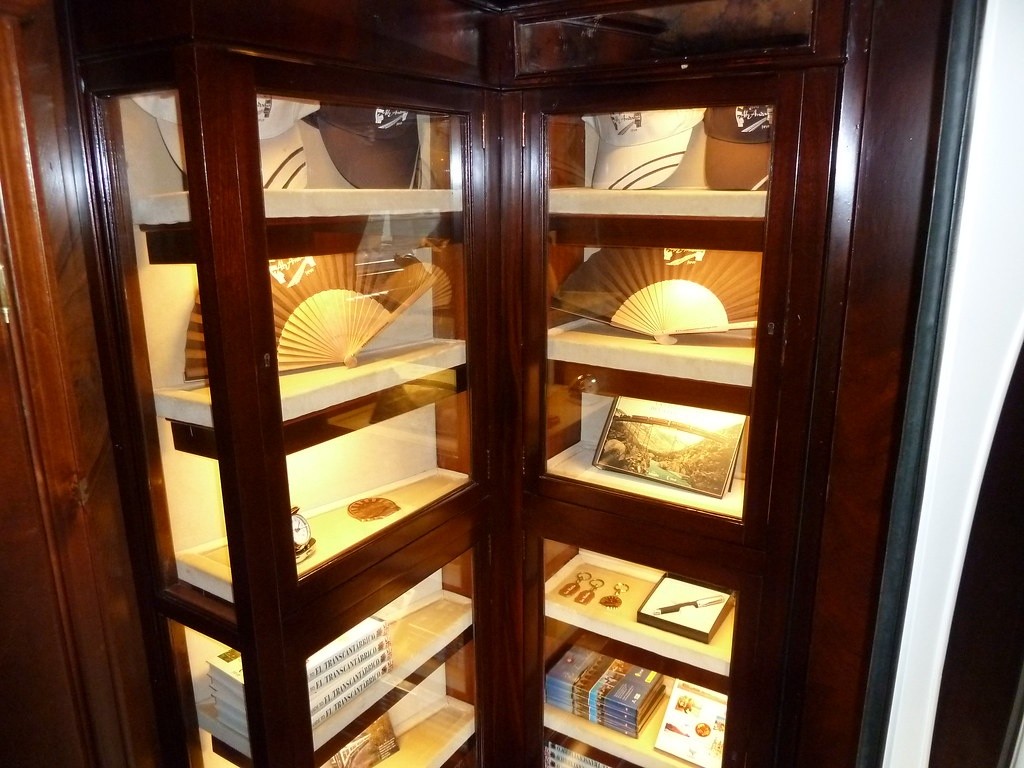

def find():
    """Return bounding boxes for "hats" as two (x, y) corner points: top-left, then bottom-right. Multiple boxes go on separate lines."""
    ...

(317, 102), (420, 190)
(705, 104), (773, 192)
(592, 108), (707, 190)
(256, 95), (321, 190)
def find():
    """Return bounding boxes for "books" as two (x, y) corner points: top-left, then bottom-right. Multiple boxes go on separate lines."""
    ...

(207, 614), (394, 738)
(544, 644), (668, 739)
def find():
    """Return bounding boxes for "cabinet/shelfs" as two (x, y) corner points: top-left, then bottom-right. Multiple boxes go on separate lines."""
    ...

(50, 0), (955, 767)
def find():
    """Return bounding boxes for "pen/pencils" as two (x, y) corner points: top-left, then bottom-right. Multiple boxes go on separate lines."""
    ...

(654, 594), (725, 617)
